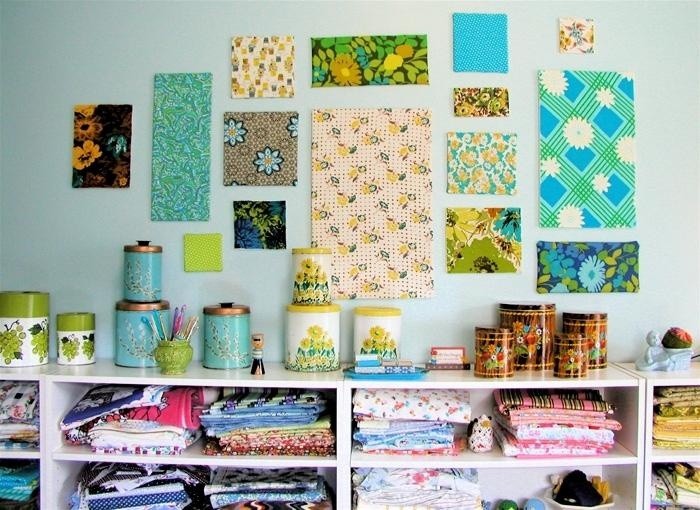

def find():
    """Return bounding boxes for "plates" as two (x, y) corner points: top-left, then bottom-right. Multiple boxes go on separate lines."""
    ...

(544, 486), (618, 510)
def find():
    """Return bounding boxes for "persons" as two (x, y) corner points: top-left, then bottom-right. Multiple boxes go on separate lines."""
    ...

(249, 333), (265, 375)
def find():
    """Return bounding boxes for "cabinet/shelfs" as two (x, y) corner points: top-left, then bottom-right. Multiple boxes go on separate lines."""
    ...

(612, 362), (699, 509)
(341, 362), (646, 508)
(0, 365), (44, 508)
(43, 363), (344, 508)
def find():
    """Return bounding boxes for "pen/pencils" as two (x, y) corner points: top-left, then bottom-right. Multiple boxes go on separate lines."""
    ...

(141, 304), (201, 342)
